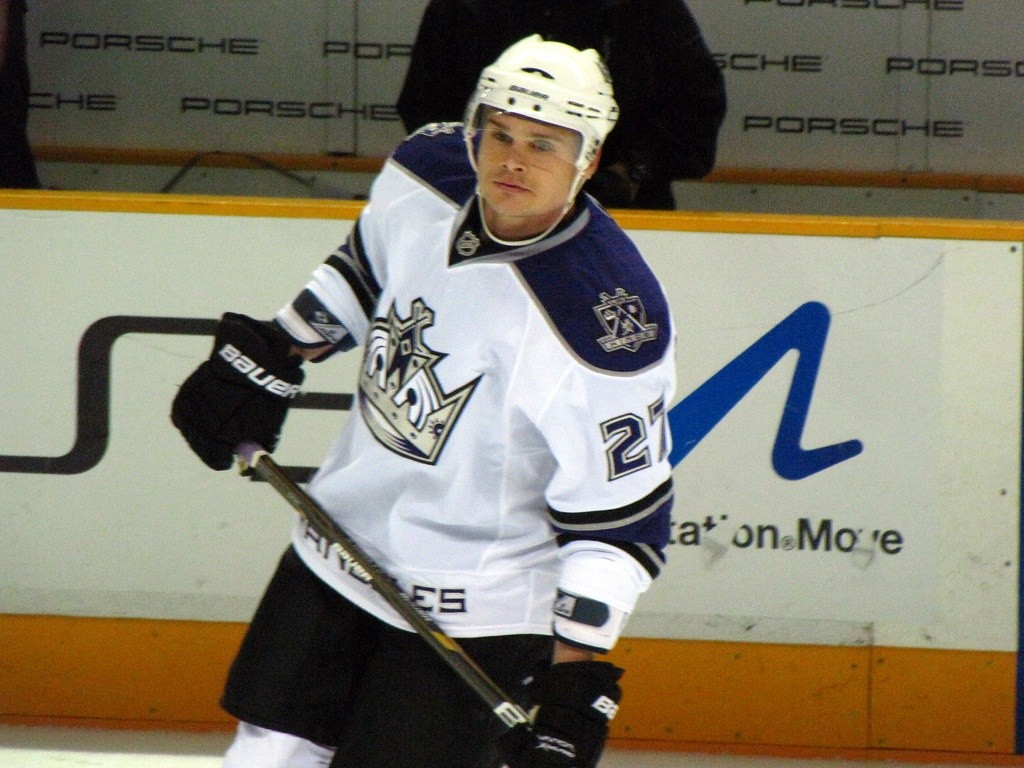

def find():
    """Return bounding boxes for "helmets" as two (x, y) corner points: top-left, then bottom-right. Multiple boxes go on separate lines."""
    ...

(464, 34), (619, 174)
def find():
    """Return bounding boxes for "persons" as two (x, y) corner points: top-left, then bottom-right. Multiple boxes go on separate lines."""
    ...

(170, 34), (677, 768)
(395, 0), (729, 211)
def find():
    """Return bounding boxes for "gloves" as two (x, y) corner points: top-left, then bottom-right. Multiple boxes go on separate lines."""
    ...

(174, 310), (306, 468)
(501, 659), (625, 768)
